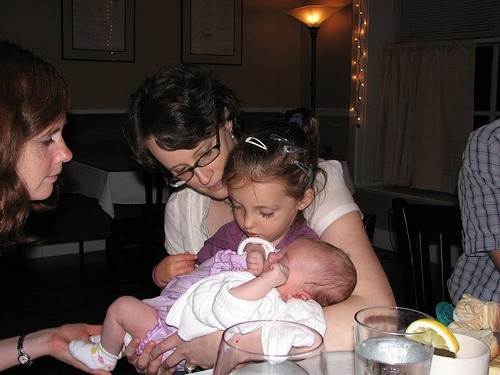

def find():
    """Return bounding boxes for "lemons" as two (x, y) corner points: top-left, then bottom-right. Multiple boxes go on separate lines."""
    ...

(405, 318), (460, 353)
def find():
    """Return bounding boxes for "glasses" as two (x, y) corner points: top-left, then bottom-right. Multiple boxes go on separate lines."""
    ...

(159, 128), (222, 190)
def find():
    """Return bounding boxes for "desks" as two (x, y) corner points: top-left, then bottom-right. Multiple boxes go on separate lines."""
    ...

(67, 158), (170, 271)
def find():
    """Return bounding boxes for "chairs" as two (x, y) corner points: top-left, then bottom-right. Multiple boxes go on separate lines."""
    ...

(110, 168), (171, 296)
(392, 198), (463, 318)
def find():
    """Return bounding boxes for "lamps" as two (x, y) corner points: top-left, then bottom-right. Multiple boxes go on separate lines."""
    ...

(284, 5), (345, 115)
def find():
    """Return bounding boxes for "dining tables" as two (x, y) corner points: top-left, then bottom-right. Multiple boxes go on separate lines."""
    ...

(186, 351), (500, 375)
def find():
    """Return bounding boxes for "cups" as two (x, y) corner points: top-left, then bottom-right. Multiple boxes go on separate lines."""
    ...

(354, 306), (437, 375)
(430, 332), (491, 375)
(212, 319), (329, 375)
